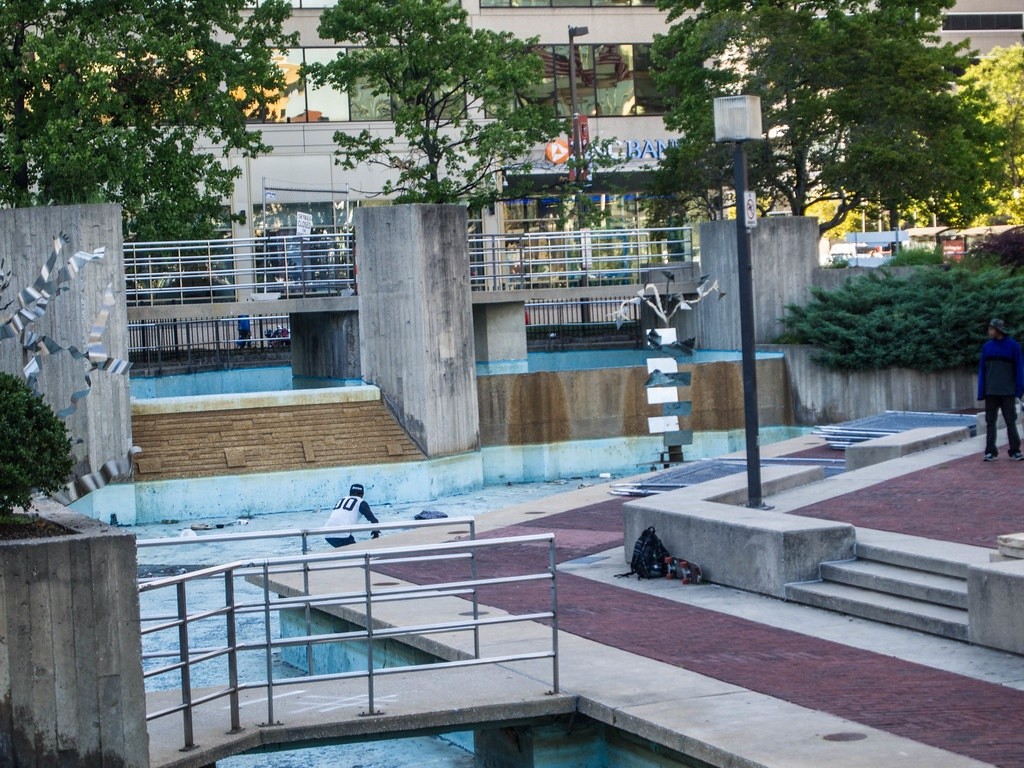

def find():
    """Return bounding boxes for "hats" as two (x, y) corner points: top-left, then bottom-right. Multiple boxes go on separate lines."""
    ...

(990, 318), (1009, 334)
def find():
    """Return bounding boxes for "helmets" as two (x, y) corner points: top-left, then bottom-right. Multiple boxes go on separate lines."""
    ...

(349, 484), (363, 496)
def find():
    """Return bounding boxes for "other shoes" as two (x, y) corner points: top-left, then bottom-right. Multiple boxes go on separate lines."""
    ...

(983, 453), (998, 461)
(1010, 453), (1024, 460)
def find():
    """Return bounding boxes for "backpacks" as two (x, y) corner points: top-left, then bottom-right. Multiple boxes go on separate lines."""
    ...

(631, 527), (670, 579)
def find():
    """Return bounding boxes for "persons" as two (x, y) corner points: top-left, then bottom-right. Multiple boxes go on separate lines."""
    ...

(977, 319), (1024, 461)
(323, 484), (381, 548)
